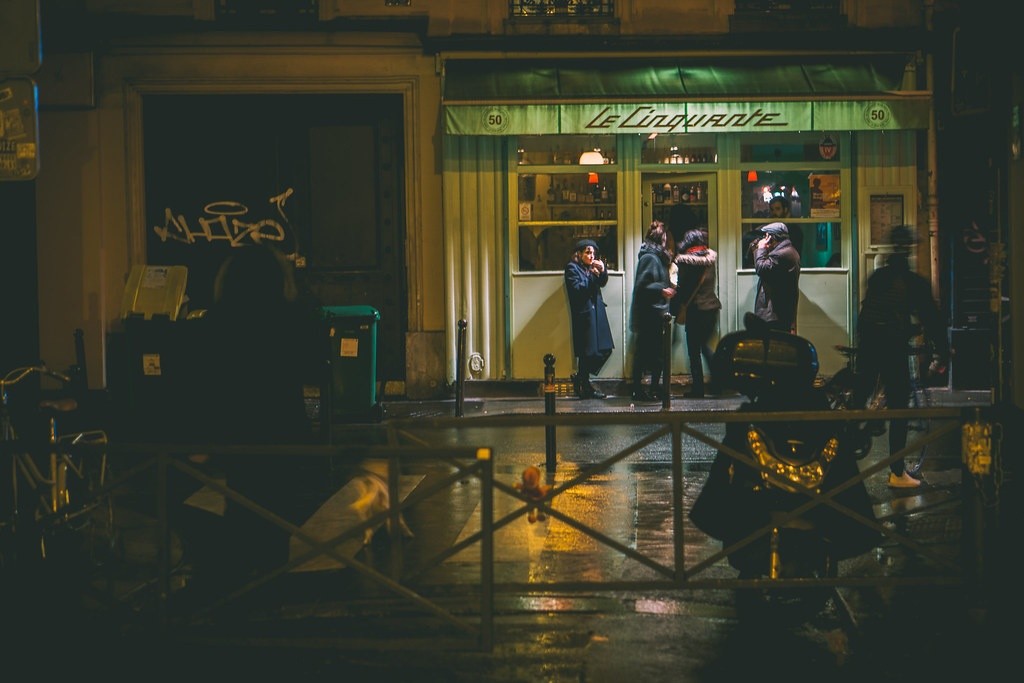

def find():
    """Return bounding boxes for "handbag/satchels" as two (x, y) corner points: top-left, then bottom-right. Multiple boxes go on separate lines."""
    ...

(675, 303), (686, 325)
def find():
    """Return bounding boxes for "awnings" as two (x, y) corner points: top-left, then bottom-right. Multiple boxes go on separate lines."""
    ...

(441, 58), (929, 135)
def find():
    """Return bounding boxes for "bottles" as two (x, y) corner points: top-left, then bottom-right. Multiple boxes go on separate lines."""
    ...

(546, 146), (712, 270)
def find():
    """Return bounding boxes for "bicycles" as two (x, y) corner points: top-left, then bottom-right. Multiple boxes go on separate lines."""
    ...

(0, 363), (119, 581)
(819, 340), (956, 478)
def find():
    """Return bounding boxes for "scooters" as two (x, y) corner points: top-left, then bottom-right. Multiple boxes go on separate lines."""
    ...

(687, 310), (886, 629)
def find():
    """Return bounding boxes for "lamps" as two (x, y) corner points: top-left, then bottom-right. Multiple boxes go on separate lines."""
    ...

(748, 171), (757, 182)
(579, 135), (604, 166)
(588, 173), (600, 184)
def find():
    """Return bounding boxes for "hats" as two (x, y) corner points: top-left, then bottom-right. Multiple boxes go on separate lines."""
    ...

(761, 222), (788, 236)
(577, 238), (599, 250)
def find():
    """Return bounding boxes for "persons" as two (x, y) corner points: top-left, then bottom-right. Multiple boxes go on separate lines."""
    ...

(669, 230), (722, 399)
(750, 223), (801, 333)
(564, 239), (615, 399)
(629, 221), (675, 402)
(857, 227), (947, 487)
(770, 197), (802, 252)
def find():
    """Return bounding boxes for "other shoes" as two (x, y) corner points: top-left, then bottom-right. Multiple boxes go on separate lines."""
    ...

(570, 372), (582, 395)
(631, 391), (653, 401)
(650, 391), (663, 400)
(889, 471), (923, 488)
(578, 389), (605, 399)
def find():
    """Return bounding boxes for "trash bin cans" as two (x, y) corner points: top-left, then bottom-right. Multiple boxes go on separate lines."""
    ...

(107, 262), (209, 445)
(315, 304), (381, 422)
